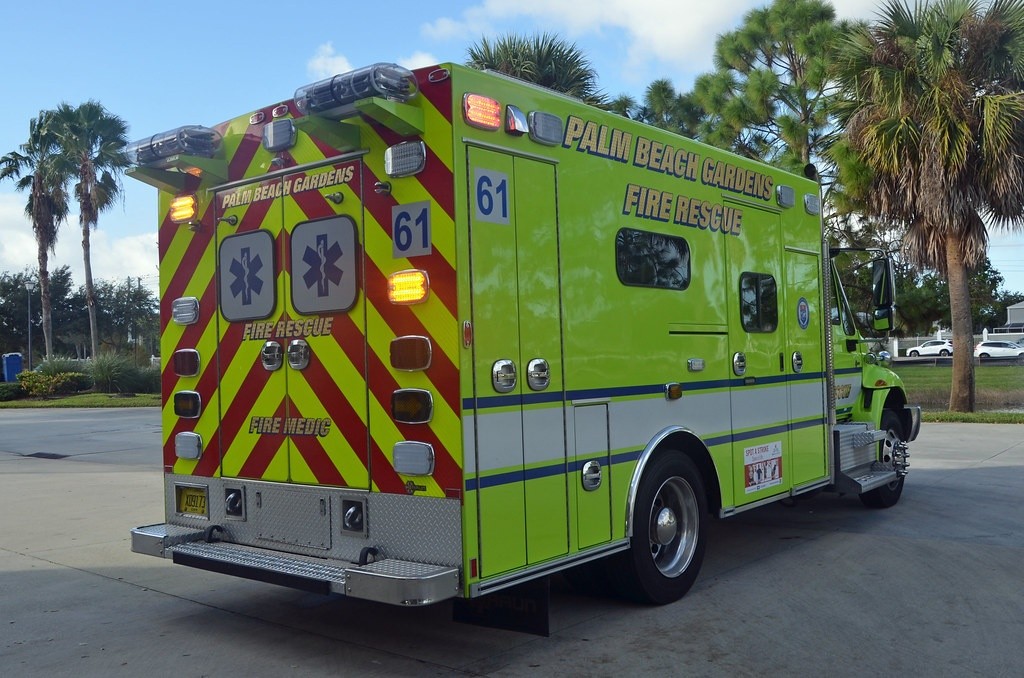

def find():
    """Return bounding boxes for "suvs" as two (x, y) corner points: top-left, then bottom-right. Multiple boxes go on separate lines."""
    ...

(905, 340), (953, 357)
(973, 341), (1024, 362)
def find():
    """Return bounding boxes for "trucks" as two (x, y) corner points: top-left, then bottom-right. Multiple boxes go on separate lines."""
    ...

(123, 61), (923, 636)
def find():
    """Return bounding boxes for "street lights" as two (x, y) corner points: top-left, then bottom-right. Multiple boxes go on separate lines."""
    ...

(25, 281), (36, 370)
